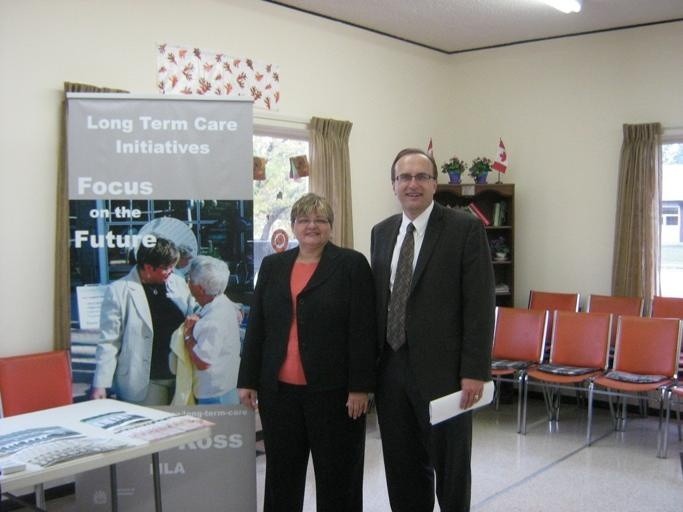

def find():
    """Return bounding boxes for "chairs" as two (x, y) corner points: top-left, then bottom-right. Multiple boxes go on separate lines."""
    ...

(519, 309), (617, 432)
(643, 294), (683, 371)
(528, 290), (581, 366)
(586, 294), (646, 418)
(0, 349), (75, 419)
(490, 306), (550, 432)
(586, 314), (682, 460)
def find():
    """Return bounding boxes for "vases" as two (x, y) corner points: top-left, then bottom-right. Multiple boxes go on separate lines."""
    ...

(448, 170), (462, 184)
(474, 172), (488, 184)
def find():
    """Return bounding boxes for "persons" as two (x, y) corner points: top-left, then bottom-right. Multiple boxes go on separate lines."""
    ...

(184, 256), (241, 406)
(90, 236), (243, 400)
(236, 194), (374, 512)
(368, 149), (494, 512)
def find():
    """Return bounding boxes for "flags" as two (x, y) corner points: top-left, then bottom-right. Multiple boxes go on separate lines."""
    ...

(427, 136), (433, 158)
(492, 136), (507, 174)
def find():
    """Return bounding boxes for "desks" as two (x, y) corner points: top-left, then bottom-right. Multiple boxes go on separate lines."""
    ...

(0, 397), (213, 509)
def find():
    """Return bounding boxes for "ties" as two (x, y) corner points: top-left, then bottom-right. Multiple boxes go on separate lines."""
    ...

(386, 222), (415, 351)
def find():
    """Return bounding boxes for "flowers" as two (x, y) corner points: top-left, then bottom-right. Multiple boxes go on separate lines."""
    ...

(441, 158), (468, 174)
(468, 157), (493, 175)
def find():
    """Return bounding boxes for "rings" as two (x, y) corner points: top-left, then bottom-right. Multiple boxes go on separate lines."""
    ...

(475, 395), (479, 399)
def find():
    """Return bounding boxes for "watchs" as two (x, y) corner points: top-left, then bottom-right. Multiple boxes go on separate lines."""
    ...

(184, 335), (192, 342)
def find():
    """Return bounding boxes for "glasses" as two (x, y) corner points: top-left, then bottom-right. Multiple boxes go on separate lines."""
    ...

(294, 216), (331, 226)
(395, 173), (436, 184)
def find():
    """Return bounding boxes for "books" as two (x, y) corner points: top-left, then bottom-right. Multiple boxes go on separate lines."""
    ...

(494, 283), (509, 293)
(494, 202), (506, 225)
(447, 202), (490, 226)
(430, 381), (495, 426)
(1, 460), (26, 477)
(77, 410), (217, 447)
(1, 424), (133, 469)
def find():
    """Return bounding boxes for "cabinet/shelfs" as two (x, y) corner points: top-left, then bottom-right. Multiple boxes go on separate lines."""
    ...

(432, 183), (517, 402)
